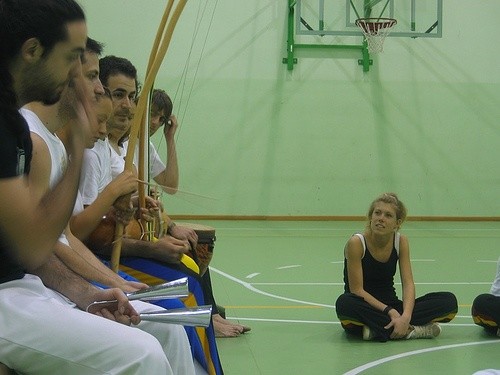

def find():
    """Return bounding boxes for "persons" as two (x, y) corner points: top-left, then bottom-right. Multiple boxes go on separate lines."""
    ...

(336, 192), (458, 342)
(471, 254), (500, 338)
(0, 0), (253, 375)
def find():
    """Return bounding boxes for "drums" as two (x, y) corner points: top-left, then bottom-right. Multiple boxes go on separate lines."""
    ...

(174, 223), (217, 277)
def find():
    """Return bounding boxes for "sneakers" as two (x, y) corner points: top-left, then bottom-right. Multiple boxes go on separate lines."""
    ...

(362, 326), (375, 340)
(404, 323), (441, 339)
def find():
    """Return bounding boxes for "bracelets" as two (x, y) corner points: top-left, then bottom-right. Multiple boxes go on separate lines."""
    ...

(383, 305), (394, 316)
(167, 222), (176, 235)
